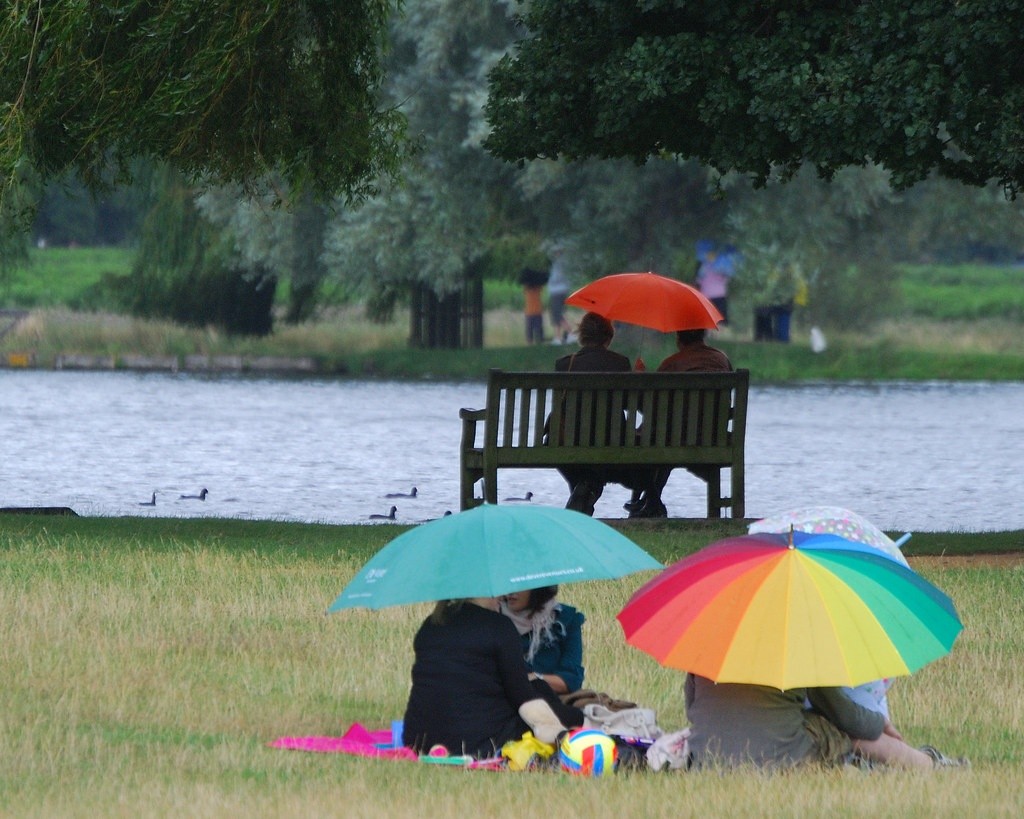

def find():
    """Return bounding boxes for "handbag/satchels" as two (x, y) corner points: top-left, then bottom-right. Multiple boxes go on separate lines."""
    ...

(542, 411), (564, 446)
(582, 704), (661, 741)
(645, 728), (693, 771)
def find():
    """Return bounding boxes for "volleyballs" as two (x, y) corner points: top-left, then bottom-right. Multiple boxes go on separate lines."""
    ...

(558, 728), (618, 780)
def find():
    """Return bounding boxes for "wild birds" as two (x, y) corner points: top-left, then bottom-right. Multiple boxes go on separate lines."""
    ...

(369, 506), (399, 520)
(385, 487), (418, 498)
(504, 492), (534, 501)
(180, 489), (208, 501)
(139, 492), (157, 506)
(417, 511), (452, 522)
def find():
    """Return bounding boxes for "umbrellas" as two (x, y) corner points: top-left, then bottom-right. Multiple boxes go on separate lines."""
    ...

(328, 480), (667, 616)
(616, 533), (966, 692)
(749, 502), (915, 569)
(562, 257), (726, 359)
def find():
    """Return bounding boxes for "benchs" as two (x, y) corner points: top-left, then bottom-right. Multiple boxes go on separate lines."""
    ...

(459, 367), (755, 519)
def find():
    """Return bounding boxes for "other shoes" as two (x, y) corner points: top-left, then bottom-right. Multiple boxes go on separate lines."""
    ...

(918, 745), (971, 770)
(564, 484), (597, 517)
(623, 496), (667, 518)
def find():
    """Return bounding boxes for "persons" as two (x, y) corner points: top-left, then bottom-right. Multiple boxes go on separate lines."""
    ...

(524, 245), (579, 345)
(695, 250), (732, 325)
(624, 329), (735, 518)
(401, 585), (655, 770)
(684, 672), (971, 773)
(543, 311), (639, 520)
